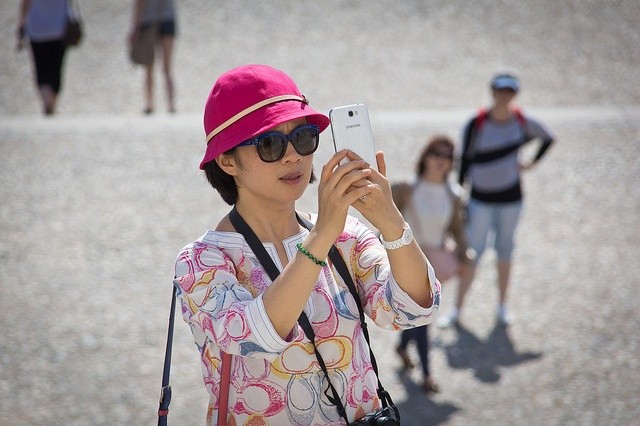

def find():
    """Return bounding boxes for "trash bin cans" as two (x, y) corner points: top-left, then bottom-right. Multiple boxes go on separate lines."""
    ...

(379, 223), (414, 250)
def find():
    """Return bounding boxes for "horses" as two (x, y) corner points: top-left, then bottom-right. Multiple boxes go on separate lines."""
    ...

(329, 103), (378, 172)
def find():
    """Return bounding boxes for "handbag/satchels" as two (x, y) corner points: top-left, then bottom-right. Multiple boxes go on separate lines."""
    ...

(296, 241), (326, 266)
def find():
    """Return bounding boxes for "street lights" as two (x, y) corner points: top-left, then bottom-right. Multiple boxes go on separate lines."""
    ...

(424, 247), (459, 283)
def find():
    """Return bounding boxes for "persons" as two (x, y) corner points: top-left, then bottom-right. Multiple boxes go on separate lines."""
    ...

(15, 1), (77, 116)
(173, 63), (442, 426)
(390, 134), (473, 392)
(133, 1), (181, 114)
(435, 70), (554, 327)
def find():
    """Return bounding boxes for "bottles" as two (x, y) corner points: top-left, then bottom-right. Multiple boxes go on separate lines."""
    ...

(199, 65), (331, 170)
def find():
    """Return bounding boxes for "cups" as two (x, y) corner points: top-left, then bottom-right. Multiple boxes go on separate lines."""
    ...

(237, 123), (320, 162)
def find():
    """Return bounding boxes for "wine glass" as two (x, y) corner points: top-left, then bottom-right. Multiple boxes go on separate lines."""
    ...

(359, 195), (367, 203)
